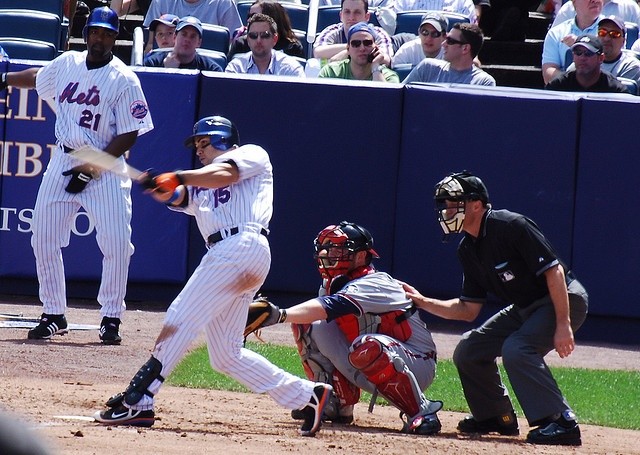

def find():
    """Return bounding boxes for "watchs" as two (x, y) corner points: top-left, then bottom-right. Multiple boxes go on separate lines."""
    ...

(372, 67), (382, 73)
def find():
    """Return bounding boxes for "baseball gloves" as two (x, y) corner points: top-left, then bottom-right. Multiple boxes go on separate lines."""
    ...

(244, 294), (280, 338)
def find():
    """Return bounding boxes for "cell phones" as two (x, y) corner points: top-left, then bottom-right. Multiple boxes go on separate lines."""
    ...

(367, 46), (379, 63)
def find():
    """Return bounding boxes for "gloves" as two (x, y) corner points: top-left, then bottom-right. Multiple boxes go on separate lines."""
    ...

(62, 162), (94, 194)
(148, 171), (183, 193)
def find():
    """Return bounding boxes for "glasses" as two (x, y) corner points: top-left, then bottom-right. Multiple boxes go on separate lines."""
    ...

(350, 40), (373, 48)
(420, 29), (441, 38)
(246, 31), (277, 39)
(571, 49), (601, 57)
(247, 13), (256, 20)
(598, 27), (624, 39)
(445, 36), (464, 46)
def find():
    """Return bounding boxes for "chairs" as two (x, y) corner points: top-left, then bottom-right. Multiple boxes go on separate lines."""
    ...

(197, 23), (240, 67)
(280, 2), (341, 67)
(396, 10), (425, 36)
(1, 0), (64, 62)
(443, 12), (470, 34)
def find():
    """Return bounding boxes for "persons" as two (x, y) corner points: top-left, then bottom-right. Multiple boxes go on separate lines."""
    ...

(0, 6), (154, 344)
(392, 11), (481, 69)
(95, 116), (333, 435)
(108, 0), (152, 17)
(567, 15), (640, 82)
(544, 35), (630, 93)
(401, 23), (496, 87)
(247, 221), (440, 434)
(228, 0), (304, 57)
(313, 0), (393, 65)
(470, 0), (491, 23)
(224, 13), (306, 77)
(551, 0), (639, 27)
(317, 23), (400, 83)
(149, 14), (180, 48)
(542, 0), (627, 84)
(390, 0), (477, 23)
(142, 0), (243, 38)
(144, 16), (223, 72)
(395, 171), (589, 445)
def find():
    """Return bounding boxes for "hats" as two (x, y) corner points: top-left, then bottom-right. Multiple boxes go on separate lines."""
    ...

(148, 14), (180, 31)
(173, 16), (204, 39)
(598, 15), (626, 34)
(418, 12), (449, 32)
(570, 34), (602, 54)
(348, 21), (376, 43)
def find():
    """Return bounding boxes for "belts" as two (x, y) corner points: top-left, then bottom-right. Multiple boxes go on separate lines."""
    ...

(565, 270), (577, 287)
(208, 226), (267, 246)
(56, 140), (75, 154)
(423, 351), (436, 363)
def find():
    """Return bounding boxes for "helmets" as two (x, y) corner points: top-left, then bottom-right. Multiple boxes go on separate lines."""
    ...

(312, 220), (379, 296)
(433, 169), (490, 248)
(184, 115), (241, 151)
(82, 5), (120, 42)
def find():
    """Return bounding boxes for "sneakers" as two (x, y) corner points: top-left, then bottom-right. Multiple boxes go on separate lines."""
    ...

(98, 316), (122, 345)
(291, 390), (353, 424)
(28, 313), (69, 340)
(298, 382), (333, 436)
(412, 412), (442, 435)
(92, 401), (156, 427)
(458, 409), (519, 436)
(526, 417), (581, 446)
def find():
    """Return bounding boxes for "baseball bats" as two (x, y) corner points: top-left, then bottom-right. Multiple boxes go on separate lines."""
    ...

(68, 145), (167, 194)
